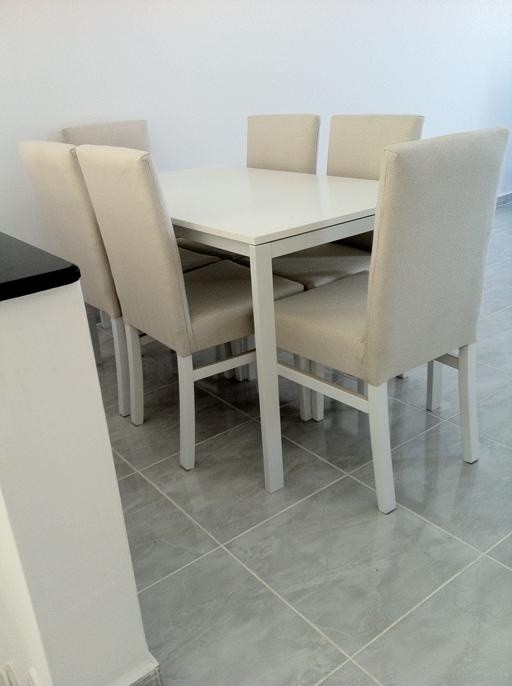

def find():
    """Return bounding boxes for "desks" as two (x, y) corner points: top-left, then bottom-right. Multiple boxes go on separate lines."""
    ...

(157, 161), (387, 494)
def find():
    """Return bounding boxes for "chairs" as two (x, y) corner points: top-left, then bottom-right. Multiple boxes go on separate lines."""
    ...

(23, 139), (145, 419)
(75, 141), (313, 474)
(63, 119), (232, 381)
(266, 124), (510, 513)
(163, 111), (320, 383)
(245, 113), (426, 424)
